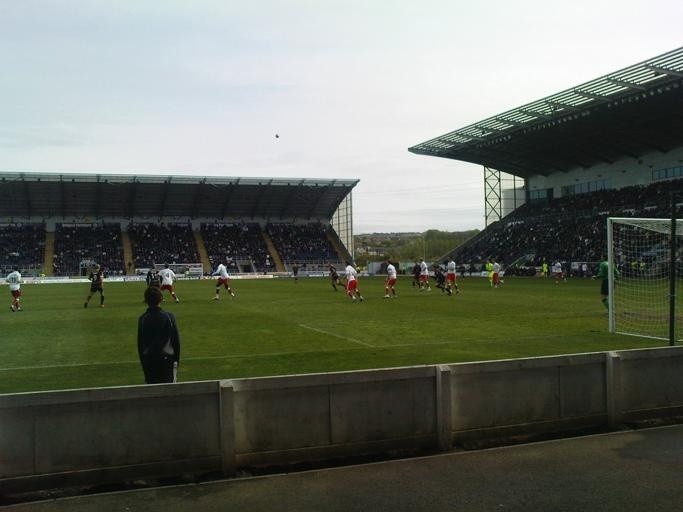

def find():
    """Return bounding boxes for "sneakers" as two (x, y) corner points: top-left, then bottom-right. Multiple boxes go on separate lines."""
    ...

(231, 292), (235, 297)
(439, 285), (461, 296)
(98, 304), (104, 307)
(488, 280), (504, 288)
(176, 298), (179, 303)
(211, 296), (219, 301)
(84, 302), (88, 308)
(10, 305), (23, 312)
(413, 285), (432, 292)
(381, 294), (396, 298)
(333, 284), (363, 302)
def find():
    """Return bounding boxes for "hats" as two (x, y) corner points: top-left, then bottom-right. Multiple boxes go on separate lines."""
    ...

(142, 286), (163, 303)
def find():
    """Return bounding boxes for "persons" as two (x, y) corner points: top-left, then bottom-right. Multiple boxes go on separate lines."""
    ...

(210, 260), (234, 301)
(338, 261), (364, 303)
(1, 222), (46, 276)
(485, 258), (493, 288)
(4, 264), (23, 312)
(326, 263), (346, 292)
(382, 258), (397, 299)
(292, 263), (299, 280)
(144, 265), (159, 289)
(82, 264), (105, 309)
(410, 261), (420, 287)
(137, 288), (179, 384)
(201, 222), (277, 273)
(553, 259), (567, 287)
(266, 223), (338, 276)
(444, 255), (460, 295)
(591, 254), (619, 314)
(155, 263), (179, 303)
(439, 176), (682, 280)
(490, 256), (503, 288)
(52, 224), (127, 279)
(129, 222), (201, 275)
(416, 257), (431, 292)
(430, 262), (452, 295)
